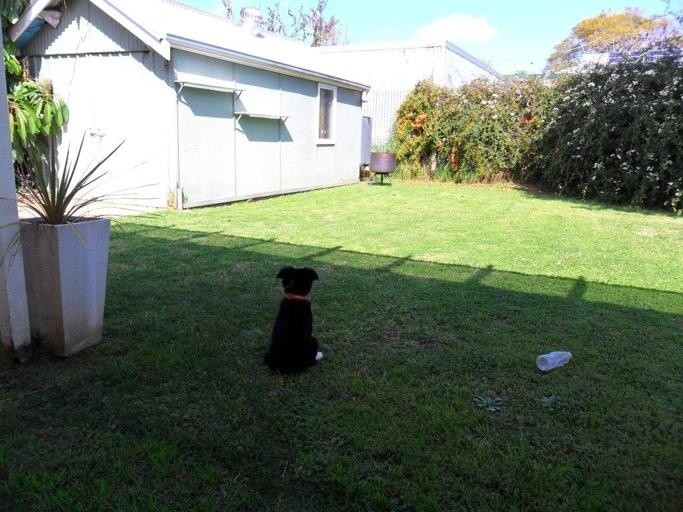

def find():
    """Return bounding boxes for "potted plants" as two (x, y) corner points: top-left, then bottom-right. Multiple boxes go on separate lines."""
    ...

(369, 141), (397, 173)
(1, 130), (167, 356)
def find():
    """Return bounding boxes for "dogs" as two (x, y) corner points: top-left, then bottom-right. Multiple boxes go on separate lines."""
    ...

(264, 264), (324, 376)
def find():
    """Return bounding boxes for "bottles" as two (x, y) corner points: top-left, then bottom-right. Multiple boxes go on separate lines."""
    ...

(534, 349), (573, 372)
